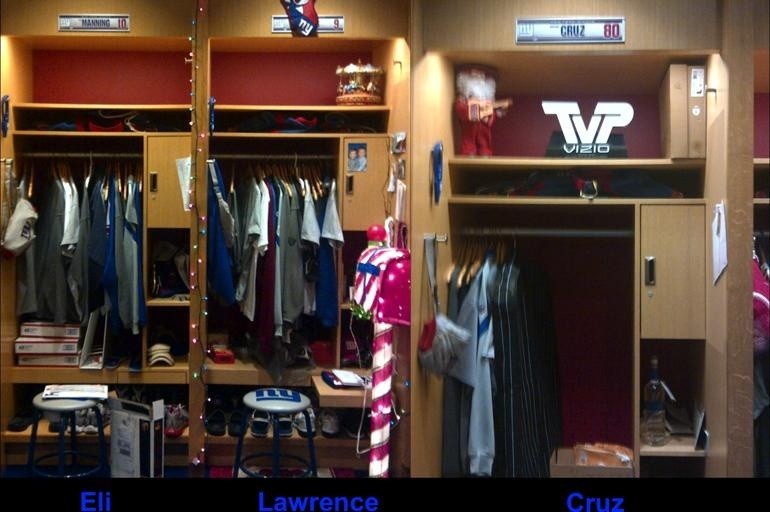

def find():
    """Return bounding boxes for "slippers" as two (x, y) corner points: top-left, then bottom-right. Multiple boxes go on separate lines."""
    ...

(204, 407), (249, 437)
(7, 405), (42, 430)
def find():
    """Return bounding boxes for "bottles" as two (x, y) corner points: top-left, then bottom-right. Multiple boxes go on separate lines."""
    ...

(0, 157), (18, 236)
(641, 358), (667, 445)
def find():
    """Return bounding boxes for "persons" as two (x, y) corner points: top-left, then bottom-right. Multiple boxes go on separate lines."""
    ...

(454, 64), (505, 155)
(280, 0), (320, 37)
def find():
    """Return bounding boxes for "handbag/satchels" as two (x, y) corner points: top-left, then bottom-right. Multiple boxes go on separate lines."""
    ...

(417, 235), (471, 373)
(1, 158), (39, 256)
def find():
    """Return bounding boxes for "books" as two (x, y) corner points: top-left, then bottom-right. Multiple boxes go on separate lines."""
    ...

(20, 321), (81, 339)
(14, 334), (83, 356)
(16, 349), (84, 368)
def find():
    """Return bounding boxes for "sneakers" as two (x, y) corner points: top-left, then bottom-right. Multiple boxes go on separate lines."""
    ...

(67, 403), (111, 433)
(166, 404), (188, 437)
(251, 408), (365, 438)
